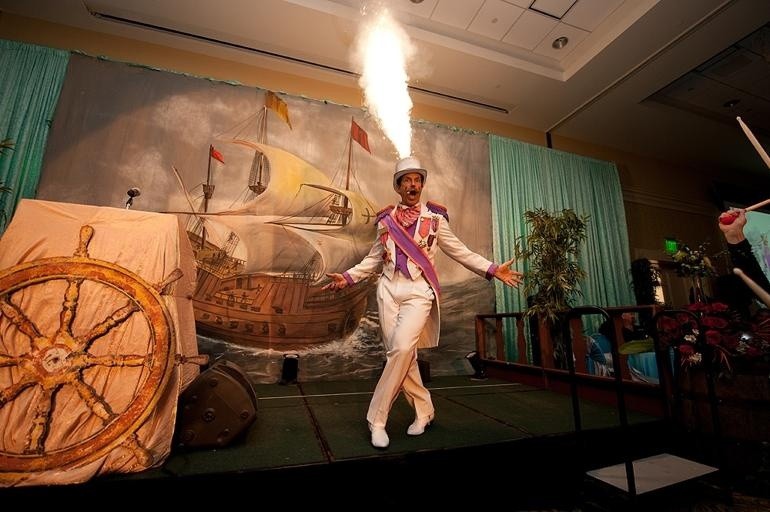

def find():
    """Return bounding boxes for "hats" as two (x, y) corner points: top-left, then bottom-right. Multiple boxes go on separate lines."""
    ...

(393, 156), (427, 188)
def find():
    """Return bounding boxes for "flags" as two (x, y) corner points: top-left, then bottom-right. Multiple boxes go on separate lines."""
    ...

(266, 90), (292, 130)
(352, 121), (371, 154)
(212, 150), (225, 163)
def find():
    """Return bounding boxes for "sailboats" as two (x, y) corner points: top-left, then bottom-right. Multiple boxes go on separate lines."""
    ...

(124, 96), (400, 352)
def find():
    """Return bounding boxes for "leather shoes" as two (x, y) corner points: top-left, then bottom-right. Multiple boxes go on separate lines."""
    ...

(368, 422), (390, 448)
(407, 413), (435, 436)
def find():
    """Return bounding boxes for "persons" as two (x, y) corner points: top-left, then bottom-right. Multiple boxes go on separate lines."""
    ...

(322, 161), (523, 447)
(718, 206), (770, 312)
